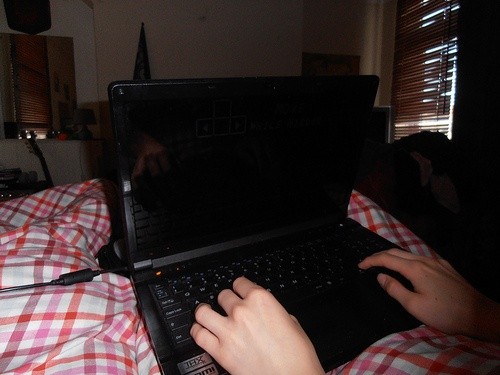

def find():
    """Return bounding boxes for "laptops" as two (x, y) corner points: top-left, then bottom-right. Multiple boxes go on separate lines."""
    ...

(107, 75), (423, 375)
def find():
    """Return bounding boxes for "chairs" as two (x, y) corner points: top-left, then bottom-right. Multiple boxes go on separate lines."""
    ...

(4, 121), (19, 139)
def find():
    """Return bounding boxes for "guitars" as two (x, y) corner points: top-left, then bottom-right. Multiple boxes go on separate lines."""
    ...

(17, 130), (54, 187)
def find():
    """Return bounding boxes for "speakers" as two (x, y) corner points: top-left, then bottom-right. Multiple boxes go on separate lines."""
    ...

(3, 0), (51, 34)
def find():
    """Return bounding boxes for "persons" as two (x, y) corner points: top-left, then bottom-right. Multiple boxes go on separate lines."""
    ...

(189, 249), (500, 375)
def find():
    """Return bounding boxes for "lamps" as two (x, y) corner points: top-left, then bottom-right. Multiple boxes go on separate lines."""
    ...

(72, 108), (97, 140)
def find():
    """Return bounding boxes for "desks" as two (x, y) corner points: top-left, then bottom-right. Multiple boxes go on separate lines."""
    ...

(0, 138), (103, 186)
